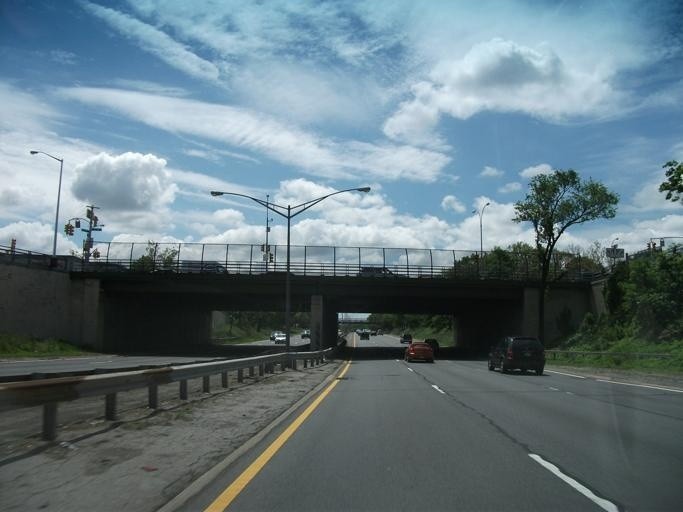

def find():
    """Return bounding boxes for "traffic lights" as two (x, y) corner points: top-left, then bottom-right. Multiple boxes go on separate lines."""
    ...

(646, 242), (651, 251)
(97, 251), (101, 258)
(64, 223), (69, 235)
(651, 243), (657, 252)
(93, 252), (96, 258)
(259, 243), (263, 250)
(270, 252), (274, 262)
(69, 225), (74, 236)
(11, 239), (16, 248)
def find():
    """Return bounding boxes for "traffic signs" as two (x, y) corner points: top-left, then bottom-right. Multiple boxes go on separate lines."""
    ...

(91, 227), (102, 231)
(82, 228), (90, 233)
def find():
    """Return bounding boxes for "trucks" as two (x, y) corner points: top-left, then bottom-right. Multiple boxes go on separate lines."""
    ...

(177, 257), (229, 275)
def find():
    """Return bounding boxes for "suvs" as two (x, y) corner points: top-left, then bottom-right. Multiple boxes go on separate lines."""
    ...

(357, 265), (407, 278)
(486, 336), (546, 375)
(399, 333), (414, 343)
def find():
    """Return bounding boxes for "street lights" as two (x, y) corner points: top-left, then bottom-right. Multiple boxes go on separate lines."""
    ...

(210, 186), (372, 367)
(30, 149), (64, 257)
(84, 205), (104, 261)
(470, 203), (491, 271)
(608, 236), (620, 267)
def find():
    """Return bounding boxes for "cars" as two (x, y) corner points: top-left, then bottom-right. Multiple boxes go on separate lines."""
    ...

(299, 328), (311, 339)
(274, 334), (288, 344)
(268, 330), (282, 340)
(350, 326), (385, 340)
(404, 341), (434, 364)
(424, 338), (441, 351)
